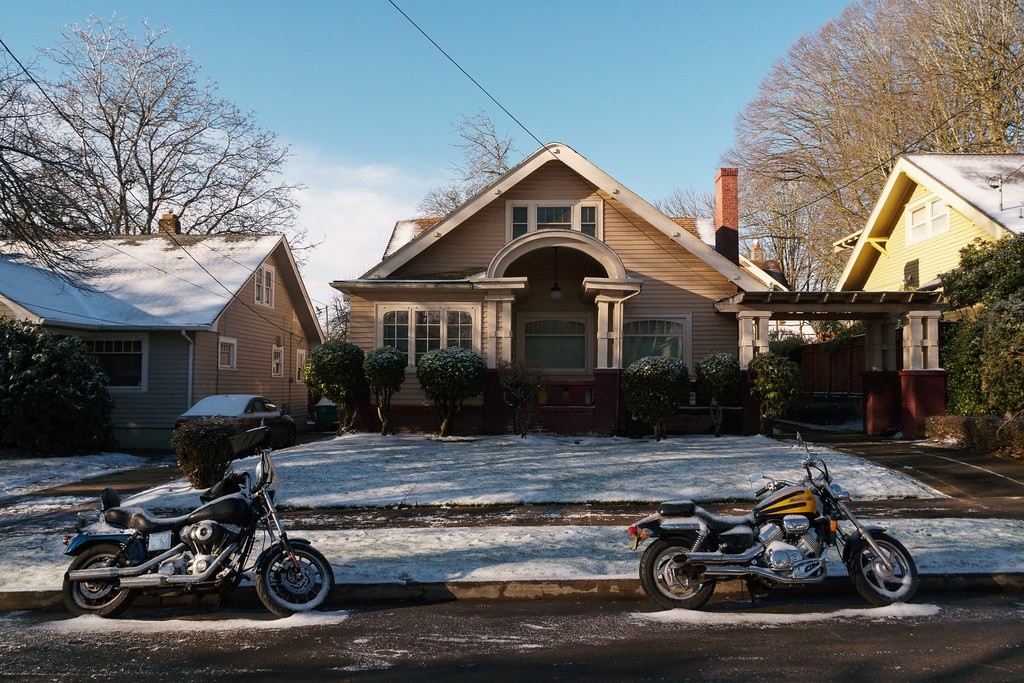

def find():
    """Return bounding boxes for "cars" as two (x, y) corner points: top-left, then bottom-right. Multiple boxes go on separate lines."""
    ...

(173, 395), (295, 456)
(312, 396), (340, 426)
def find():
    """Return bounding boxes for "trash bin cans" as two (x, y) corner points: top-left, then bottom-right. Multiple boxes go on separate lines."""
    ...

(897, 368), (947, 440)
(861, 367), (902, 436)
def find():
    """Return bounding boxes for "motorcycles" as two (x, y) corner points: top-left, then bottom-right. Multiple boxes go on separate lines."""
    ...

(62, 445), (335, 619)
(629, 431), (918, 610)
